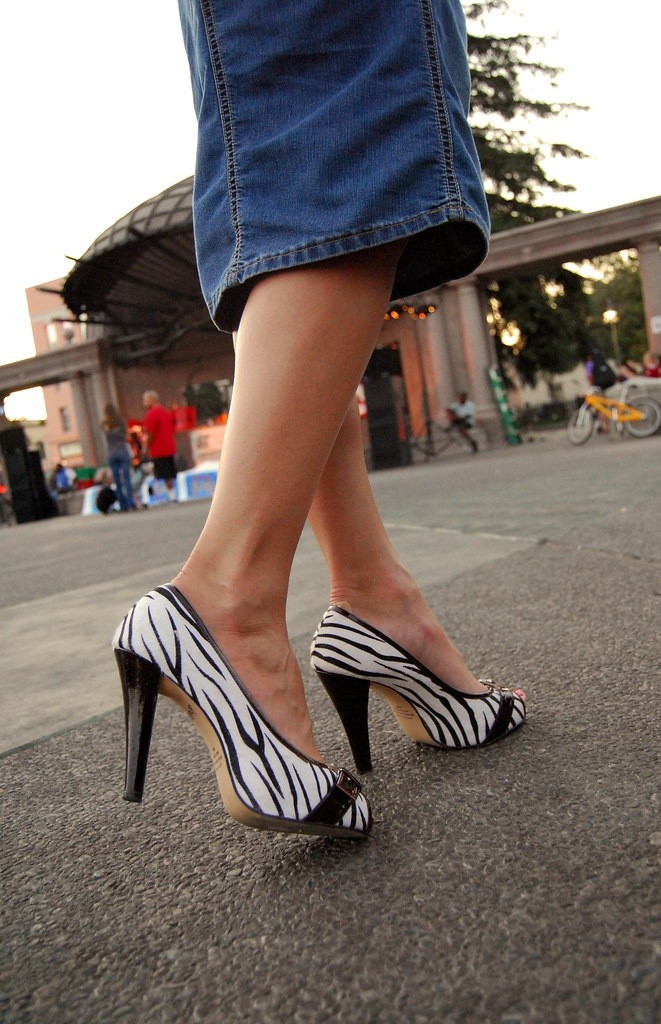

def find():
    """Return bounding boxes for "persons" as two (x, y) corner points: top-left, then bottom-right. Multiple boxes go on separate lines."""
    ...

(109, 0), (527, 836)
(104, 406), (139, 512)
(447, 391), (475, 433)
(140, 390), (176, 490)
(583, 347), (616, 433)
(56, 464), (70, 493)
(622, 349), (661, 382)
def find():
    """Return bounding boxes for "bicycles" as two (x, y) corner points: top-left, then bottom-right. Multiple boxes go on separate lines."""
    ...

(568, 376), (661, 446)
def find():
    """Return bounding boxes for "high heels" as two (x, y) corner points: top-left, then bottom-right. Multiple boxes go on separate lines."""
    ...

(111, 584), (373, 837)
(310, 604), (526, 772)
